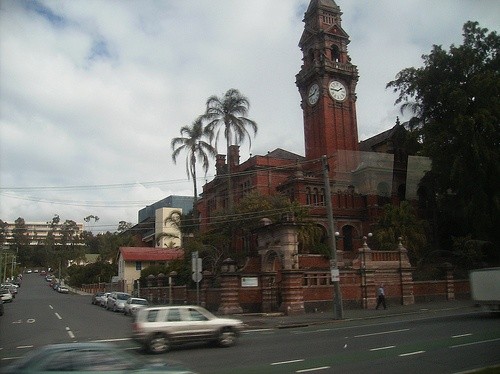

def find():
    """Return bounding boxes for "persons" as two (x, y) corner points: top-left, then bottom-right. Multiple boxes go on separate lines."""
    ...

(375, 284), (388, 310)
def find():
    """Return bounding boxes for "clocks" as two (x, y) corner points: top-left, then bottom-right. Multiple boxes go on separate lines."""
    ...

(328, 80), (347, 101)
(308, 84), (320, 104)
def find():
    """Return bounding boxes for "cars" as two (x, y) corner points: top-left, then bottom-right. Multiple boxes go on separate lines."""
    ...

(1, 343), (192, 374)
(0, 274), (23, 315)
(41, 270), (69, 293)
(26, 269), (33, 273)
(124, 298), (150, 317)
(106, 291), (133, 312)
(91, 291), (110, 307)
(33, 268), (41, 272)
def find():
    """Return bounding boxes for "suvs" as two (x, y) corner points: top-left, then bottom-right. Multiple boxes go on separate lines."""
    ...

(132, 304), (245, 355)
